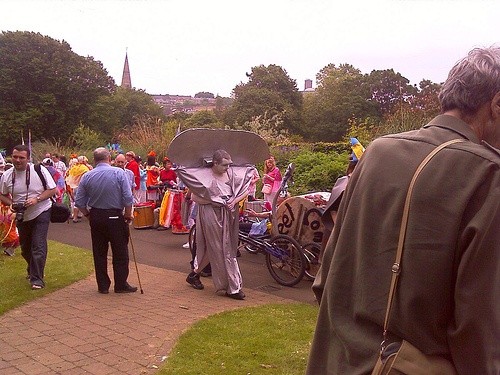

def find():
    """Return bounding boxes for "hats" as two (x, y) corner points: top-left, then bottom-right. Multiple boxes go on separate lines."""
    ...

(43, 158), (55, 166)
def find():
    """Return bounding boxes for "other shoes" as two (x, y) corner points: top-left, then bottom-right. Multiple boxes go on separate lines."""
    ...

(98, 287), (109, 294)
(26, 274), (31, 281)
(32, 284), (42, 289)
(5, 249), (15, 257)
(226, 290), (246, 299)
(73, 219), (82, 223)
(114, 285), (138, 292)
(183, 241), (193, 249)
(187, 273), (205, 290)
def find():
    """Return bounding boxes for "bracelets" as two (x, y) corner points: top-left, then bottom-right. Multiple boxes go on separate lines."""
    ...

(84, 213), (90, 217)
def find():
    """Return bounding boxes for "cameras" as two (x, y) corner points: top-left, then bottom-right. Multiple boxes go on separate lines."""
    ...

(12, 201), (27, 212)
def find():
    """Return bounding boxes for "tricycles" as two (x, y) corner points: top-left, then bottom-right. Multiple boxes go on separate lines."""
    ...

(188, 207), (325, 289)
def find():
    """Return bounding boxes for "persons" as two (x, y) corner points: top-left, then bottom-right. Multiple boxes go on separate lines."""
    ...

(243, 189), (291, 236)
(248, 168), (260, 201)
(110, 151), (196, 249)
(260, 159), (282, 209)
(43, 154), (93, 223)
(0, 145), (57, 289)
(307, 45), (500, 375)
(74, 147), (138, 294)
(171, 149), (254, 299)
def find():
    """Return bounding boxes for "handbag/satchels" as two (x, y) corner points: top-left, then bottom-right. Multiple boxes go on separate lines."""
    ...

(51, 203), (70, 224)
(261, 184), (272, 194)
(371, 338), (457, 375)
